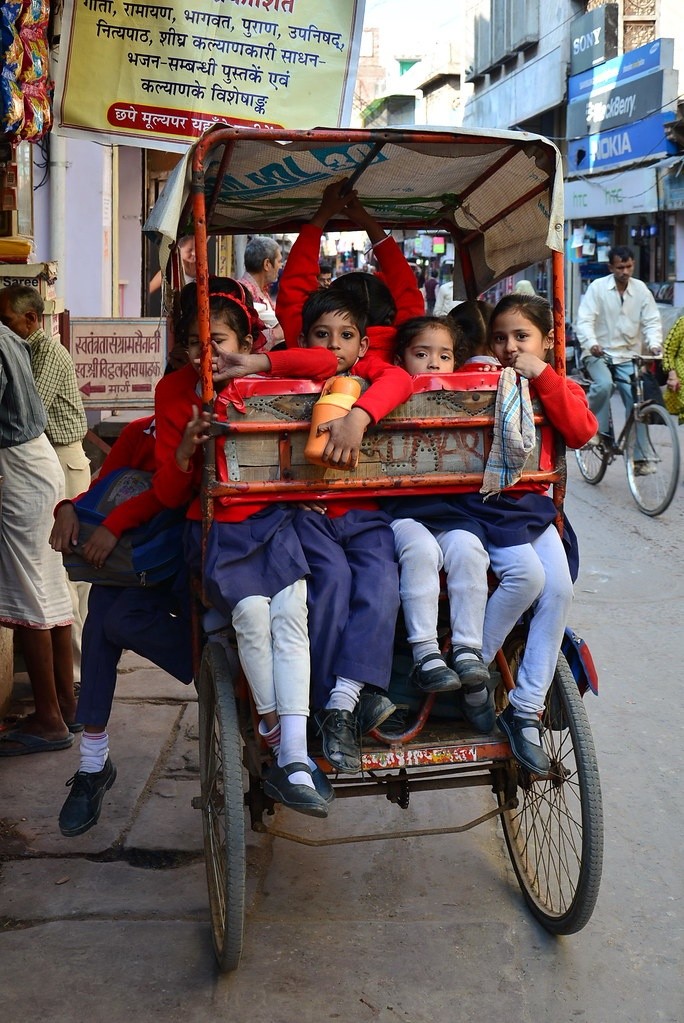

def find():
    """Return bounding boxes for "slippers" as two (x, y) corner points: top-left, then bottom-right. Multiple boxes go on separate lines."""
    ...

(0, 726), (76, 756)
(65, 719), (84, 732)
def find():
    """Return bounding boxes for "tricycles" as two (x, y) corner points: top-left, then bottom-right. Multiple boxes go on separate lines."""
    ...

(143, 126), (604, 974)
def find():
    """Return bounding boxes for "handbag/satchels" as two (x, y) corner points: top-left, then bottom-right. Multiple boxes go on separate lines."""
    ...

(61, 468), (190, 586)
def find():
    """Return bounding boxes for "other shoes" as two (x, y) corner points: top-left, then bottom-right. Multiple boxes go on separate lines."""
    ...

(599, 431), (618, 454)
(634, 461), (657, 474)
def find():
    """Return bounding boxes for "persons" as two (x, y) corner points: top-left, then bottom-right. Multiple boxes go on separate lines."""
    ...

(0, 238), (599, 839)
(150, 237), (211, 294)
(576, 247), (663, 477)
(662, 315), (684, 426)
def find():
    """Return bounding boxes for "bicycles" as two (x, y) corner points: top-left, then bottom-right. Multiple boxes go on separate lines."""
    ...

(567, 349), (680, 517)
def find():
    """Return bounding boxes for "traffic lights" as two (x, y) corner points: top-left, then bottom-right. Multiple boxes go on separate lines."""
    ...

(343, 251), (351, 261)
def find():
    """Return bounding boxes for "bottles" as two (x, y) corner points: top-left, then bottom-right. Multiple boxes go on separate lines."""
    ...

(305, 378), (368, 473)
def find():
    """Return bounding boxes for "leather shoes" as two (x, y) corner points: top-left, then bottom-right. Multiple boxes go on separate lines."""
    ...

(497, 701), (551, 777)
(313, 703), (365, 782)
(59, 755), (116, 835)
(449, 646), (489, 683)
(264, 760), (335, 818)
(468, 686), (494, 733)
(351, 689), (396, 733)
(412, 653), (462, 692)
